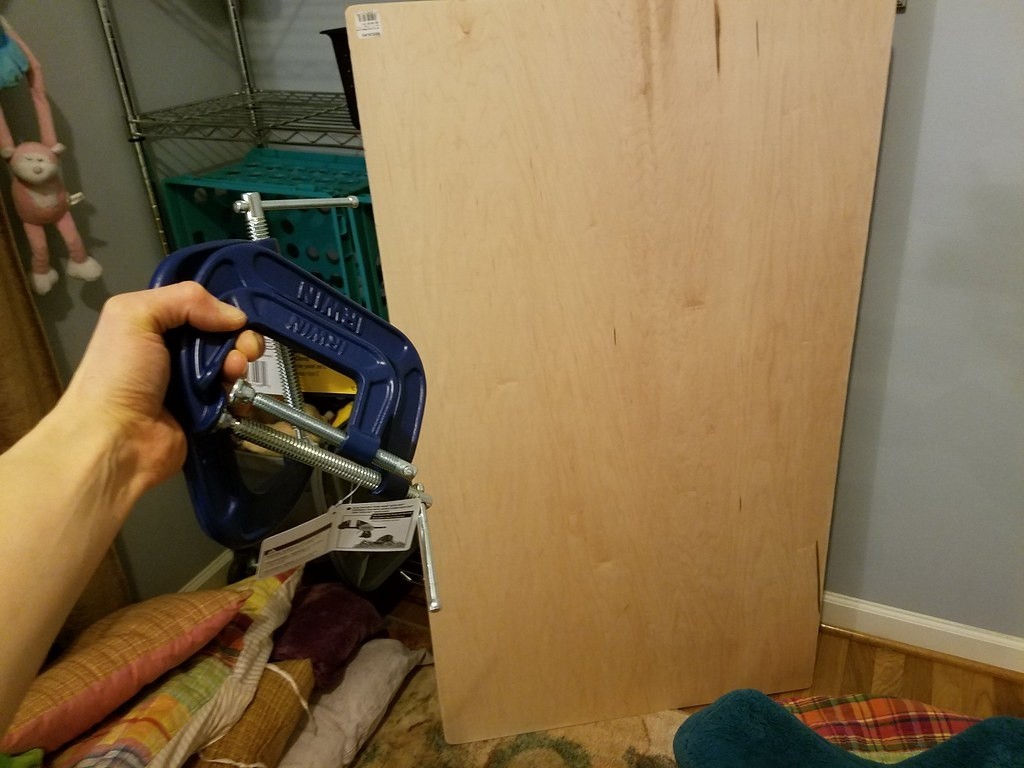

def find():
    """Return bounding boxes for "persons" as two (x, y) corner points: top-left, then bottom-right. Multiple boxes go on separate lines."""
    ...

(0, 280), (265, 743)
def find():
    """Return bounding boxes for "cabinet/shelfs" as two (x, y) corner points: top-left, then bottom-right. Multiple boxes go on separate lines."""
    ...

(93, 0), (407, 463)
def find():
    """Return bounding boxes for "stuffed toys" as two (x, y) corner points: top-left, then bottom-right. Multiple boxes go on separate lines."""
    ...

(0, 15), (103, 296)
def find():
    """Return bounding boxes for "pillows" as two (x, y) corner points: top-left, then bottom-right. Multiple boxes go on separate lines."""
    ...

(0, 564), (424, 768)
